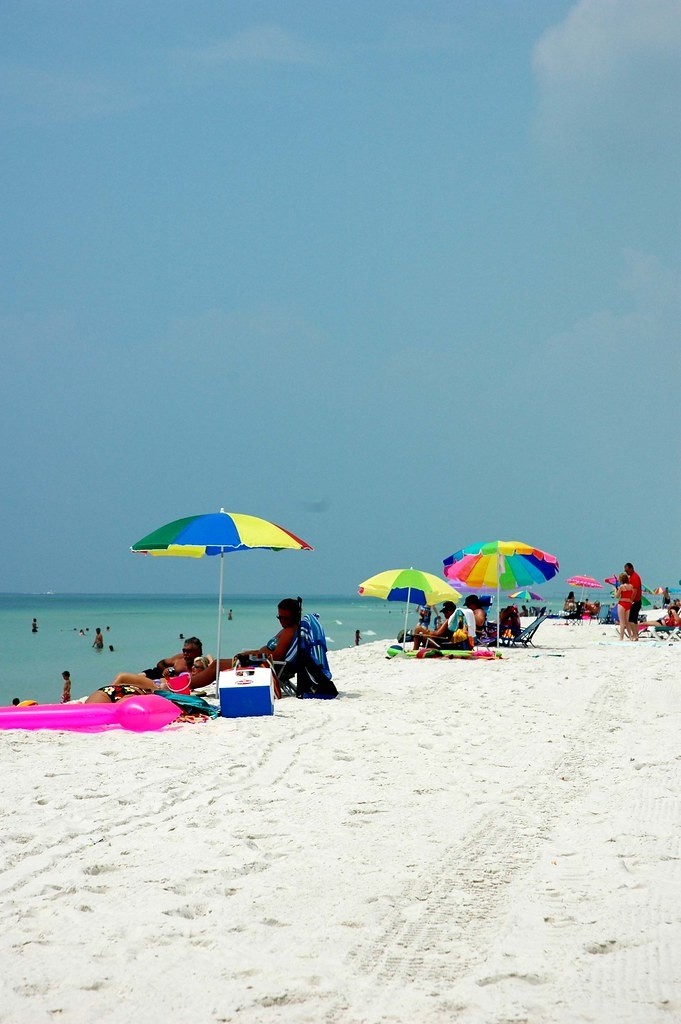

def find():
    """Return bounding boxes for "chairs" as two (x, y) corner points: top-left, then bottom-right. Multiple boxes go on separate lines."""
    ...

(413, 605), (477, 651)
(527, 600), (681, 643)
(233, 612), (339, 700)
(487, 606), (546, 647)
(484, 611), (551, 648)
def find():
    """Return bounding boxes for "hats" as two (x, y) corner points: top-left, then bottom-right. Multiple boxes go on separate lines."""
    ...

(463, 595), (478, 606)
(439, 601), (456, 612)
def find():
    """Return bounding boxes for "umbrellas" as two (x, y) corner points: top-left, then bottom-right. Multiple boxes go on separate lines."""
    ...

(566, 573), (604, 602)
(129, 507), (315, 700)
(605, 576), (681, 606)
(508, 591), (543, 605)
(358, 567), (463, 651)
(442, 540), (560, 648)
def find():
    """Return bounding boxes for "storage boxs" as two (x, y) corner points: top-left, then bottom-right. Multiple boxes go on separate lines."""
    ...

(217, 666), (274, 718)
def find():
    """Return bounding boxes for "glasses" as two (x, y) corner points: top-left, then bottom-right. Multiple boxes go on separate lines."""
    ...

(275, 613), (293, 620)
(182, 649), (193, 653)
(192, 664), (200, 669)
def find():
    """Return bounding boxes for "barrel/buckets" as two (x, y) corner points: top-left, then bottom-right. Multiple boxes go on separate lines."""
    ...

(165, 673), (191, 696)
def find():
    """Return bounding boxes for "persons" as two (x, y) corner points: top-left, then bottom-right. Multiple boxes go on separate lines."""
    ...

(32, 617), (39, 632)
(354, 630), (361, 646)
(12, 698), (20, 706)
(179, 634), (185, 639)
(413, 594), (521, 650)
(60, 626), (114, 653)
(625, 563), (642, 642)
(613, 572), (634, 641)
(228, 609), (233, 620)
(638, 587), (681, 628)
(60, 671), (72, 704)
(563, 591), (601, 616)
(86, 637), (214, 703)
(189, 599), (300, 689)
(520, 604), (552, 617)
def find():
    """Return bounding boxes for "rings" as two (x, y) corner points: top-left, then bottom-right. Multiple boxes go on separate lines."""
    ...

(166, 669), (169, 672)
